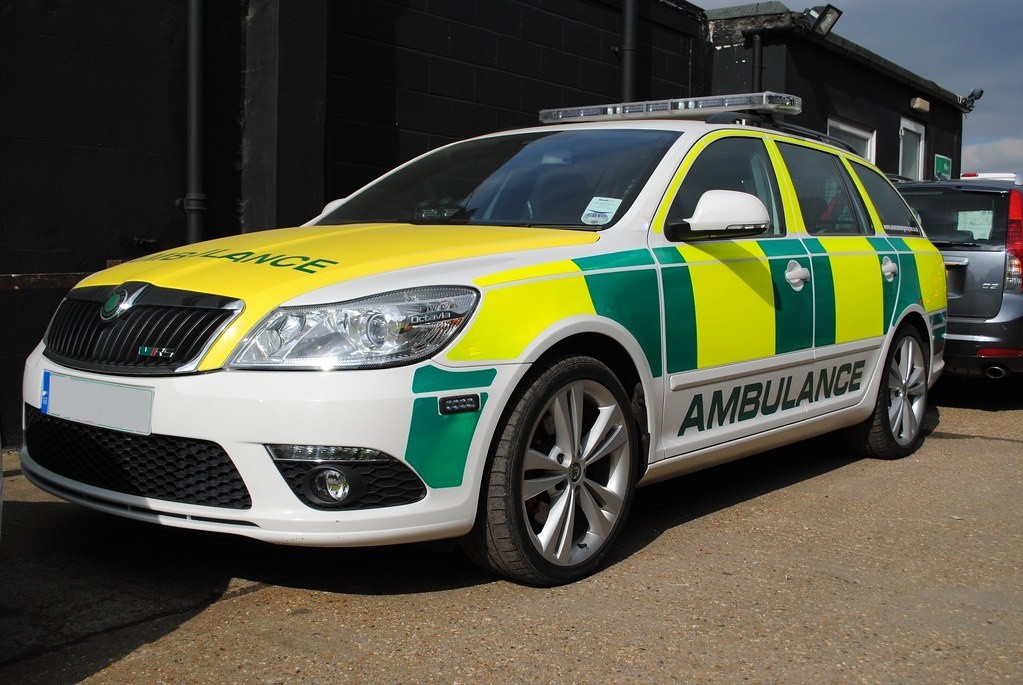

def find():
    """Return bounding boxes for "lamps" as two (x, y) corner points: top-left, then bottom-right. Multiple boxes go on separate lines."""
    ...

(792, 4), (842, 38)
(964, 88), (984, 109)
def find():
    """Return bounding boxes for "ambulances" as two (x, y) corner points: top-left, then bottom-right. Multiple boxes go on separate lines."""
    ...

(17, 92), (952, 586)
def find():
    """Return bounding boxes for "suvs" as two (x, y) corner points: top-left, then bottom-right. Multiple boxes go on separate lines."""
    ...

(819, 171), (1023, 387)
